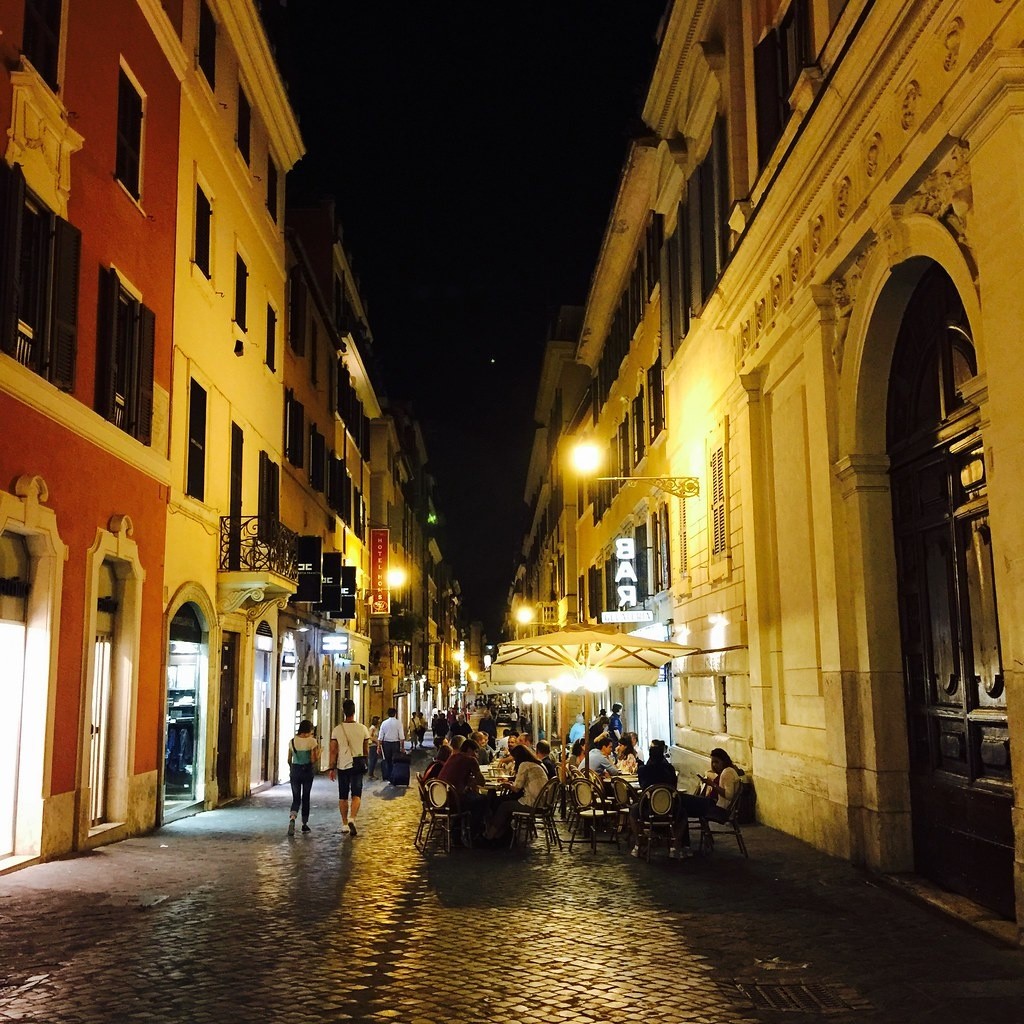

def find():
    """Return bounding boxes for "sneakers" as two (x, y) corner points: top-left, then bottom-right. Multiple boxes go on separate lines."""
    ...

(630, 844), (650, 858)
(301, 825), (311, 834)
(669, 848), (677, 858)
(336, 824), (349, 833)
(288, 820), (295, 836)
(348, 818), (357, 836)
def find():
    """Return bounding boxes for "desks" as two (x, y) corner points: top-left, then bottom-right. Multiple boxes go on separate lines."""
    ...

(479, 763), (516, 806)
(630, 781), (688, 797)
(602, 774), (639, 783)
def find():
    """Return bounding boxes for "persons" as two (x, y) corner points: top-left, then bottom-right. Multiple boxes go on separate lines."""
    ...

(287, 720), (320, 836)
(329, 700), (370, 835)
(368, 715), (382, 780)
(568, 702), (742, 859)
(424, 698), (556, 846)
(408, 711), (426, 750)
(377, 708), (406, 785)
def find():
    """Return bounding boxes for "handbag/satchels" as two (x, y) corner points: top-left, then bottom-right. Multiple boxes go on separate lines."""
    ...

(415, 728), (419, 735)
(391, 752), (411, 763)
(353, 756), (368, 775)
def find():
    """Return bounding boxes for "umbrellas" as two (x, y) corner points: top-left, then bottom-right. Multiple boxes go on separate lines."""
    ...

(477, 624), (697, 819)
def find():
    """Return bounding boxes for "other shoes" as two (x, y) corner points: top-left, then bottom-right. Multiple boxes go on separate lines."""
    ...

(369, 776), (380, 781)
(461, 830), (475, 849)
(678, 846), (693, 858)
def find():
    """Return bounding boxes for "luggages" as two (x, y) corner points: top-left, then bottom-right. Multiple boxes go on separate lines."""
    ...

(389, 762), (410, 787)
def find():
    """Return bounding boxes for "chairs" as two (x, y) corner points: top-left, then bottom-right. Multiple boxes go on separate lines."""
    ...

(414, 710), (746, 856)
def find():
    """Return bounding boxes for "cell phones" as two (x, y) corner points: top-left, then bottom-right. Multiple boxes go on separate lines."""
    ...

(696, 773), (704, 780)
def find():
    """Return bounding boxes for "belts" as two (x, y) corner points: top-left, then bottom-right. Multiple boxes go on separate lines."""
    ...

(291, 765), (307, 769)
